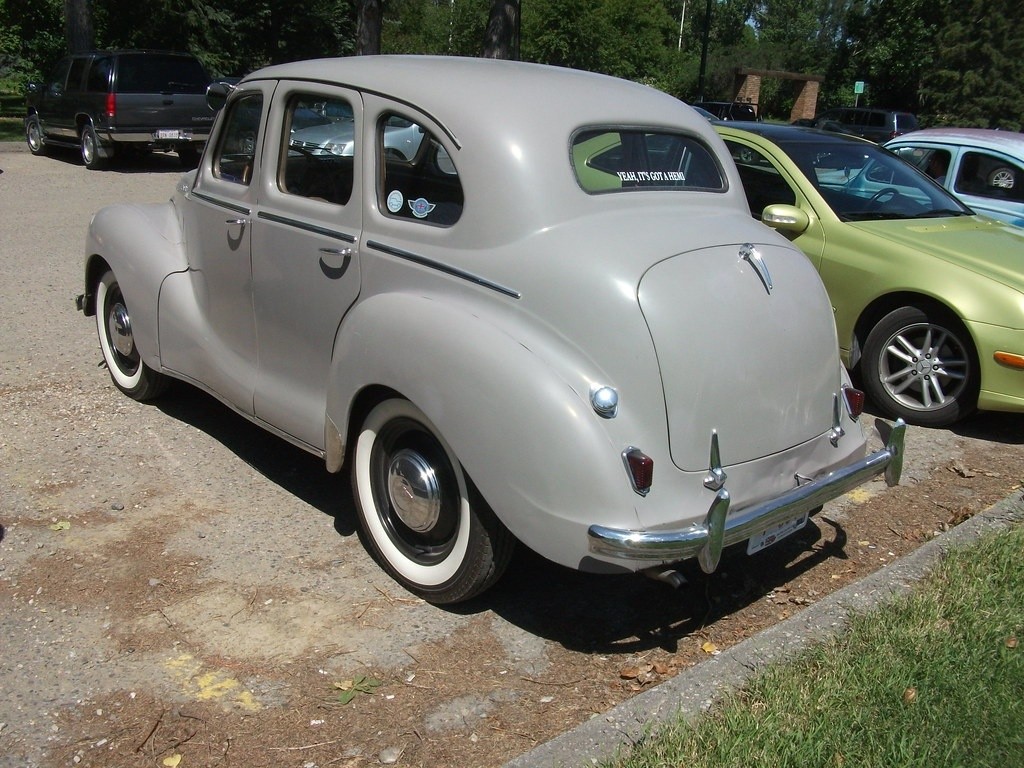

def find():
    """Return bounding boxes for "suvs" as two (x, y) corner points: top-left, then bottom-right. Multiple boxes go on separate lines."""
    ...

(21, 46), (228, 172)
(788, 106), (922, 168)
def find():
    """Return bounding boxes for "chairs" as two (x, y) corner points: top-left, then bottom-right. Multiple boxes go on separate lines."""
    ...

(771, 152), (823, 203)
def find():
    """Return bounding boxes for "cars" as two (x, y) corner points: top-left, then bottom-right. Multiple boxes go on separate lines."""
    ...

(568, 119), (1024, 429)
(814, 125), (1024, 230)
(687, 95), (764, 123)
(212, 75), (457, 178)
(72, 50), (909, 609)
